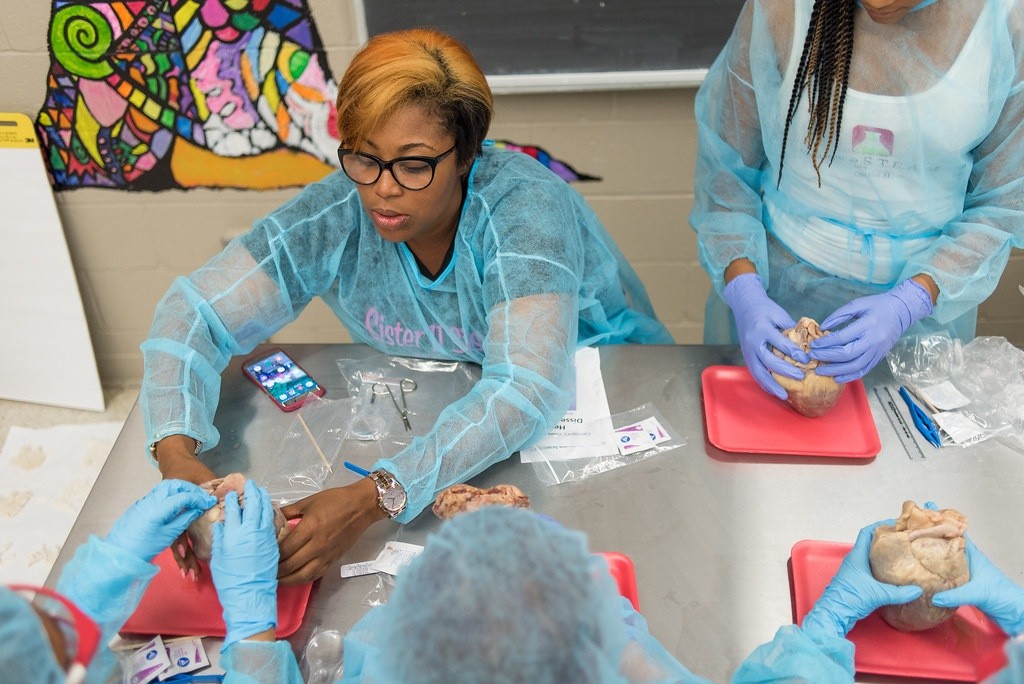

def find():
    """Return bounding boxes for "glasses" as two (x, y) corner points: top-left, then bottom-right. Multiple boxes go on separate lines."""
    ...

(336, 143), (459, 192)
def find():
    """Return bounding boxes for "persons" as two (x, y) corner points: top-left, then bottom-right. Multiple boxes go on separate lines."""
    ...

(732, 502), (1024, 683)
(140, 30), (675, 586)
(690, 0), (1024, 400)
(339, 506), (708, 683)
(0, 477), (304, 684)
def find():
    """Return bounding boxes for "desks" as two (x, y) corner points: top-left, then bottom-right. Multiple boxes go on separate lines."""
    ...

(32, 342), (1023, 684)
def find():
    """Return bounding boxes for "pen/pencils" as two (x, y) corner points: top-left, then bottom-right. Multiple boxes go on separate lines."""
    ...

(297, 413), (334, 474)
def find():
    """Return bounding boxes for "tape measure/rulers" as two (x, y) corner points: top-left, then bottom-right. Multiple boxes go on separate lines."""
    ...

(874, 386), (926, 462)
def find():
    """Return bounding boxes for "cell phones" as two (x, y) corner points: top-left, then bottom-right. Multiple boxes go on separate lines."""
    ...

(241, 346), (326, 413)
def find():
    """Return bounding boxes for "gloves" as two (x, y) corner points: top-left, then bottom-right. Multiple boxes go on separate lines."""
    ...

(807, 280), (936, 386)
(208, 478), (279, 656)
(104, 480), (215, 564)
(925, 502), (1024, 634)
(724, 272), (813, 400)
(801, 518), (922, 649)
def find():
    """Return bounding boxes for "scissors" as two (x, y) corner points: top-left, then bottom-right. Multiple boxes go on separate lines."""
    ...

(372, 378), (417, 431)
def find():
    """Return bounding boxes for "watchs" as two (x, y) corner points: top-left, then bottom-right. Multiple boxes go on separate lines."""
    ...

(369, 469), (407, 520)
